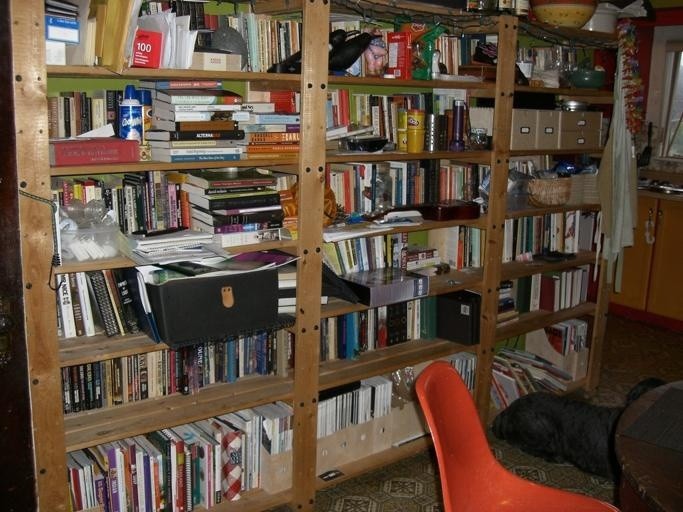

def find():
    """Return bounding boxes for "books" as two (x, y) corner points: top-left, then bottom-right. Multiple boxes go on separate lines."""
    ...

(317, 21), (477, 437)
(51, 168), (297, 267)
(43, 1), (300, 73)
(55, 251), (303, 339)
(66, 401), (293, 511)
(60, 329), (295, 413)
(48, 79), (301, 165)
(491, 209), (594, 409)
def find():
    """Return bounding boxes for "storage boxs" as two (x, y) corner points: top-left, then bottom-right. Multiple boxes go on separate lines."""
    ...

(468, 106), (604, 150)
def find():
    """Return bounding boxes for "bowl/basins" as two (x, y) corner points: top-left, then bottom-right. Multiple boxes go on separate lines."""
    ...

(530, 0), (596, 29)
(567, 70), (606, 89)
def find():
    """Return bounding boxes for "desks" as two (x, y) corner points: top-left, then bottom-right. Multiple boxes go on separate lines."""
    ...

(613, 379), (683, 512)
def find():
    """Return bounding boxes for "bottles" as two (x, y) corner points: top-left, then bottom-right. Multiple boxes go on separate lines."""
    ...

(463, 0), (529, 17)
(469, 128), (488, 150)
(412, 40), (441, 80)
(424, 114), (448, 153)
(118, 84), (153, 146)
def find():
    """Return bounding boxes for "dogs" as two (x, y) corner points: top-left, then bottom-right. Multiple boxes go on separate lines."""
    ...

(490, 376), (668, 483)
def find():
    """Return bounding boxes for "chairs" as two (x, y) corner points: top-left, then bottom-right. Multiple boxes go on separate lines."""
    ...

(414, 362), (622, 512)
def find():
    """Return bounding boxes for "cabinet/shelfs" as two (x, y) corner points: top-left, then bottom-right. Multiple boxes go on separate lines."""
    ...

(309, 1), (511, 512)
(610, 186), (683, 335)
(1, 1), (310, 512)
(481, 15), (624, 427)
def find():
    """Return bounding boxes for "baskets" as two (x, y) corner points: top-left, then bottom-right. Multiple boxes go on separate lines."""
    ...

(527, 177), (572, 208)
